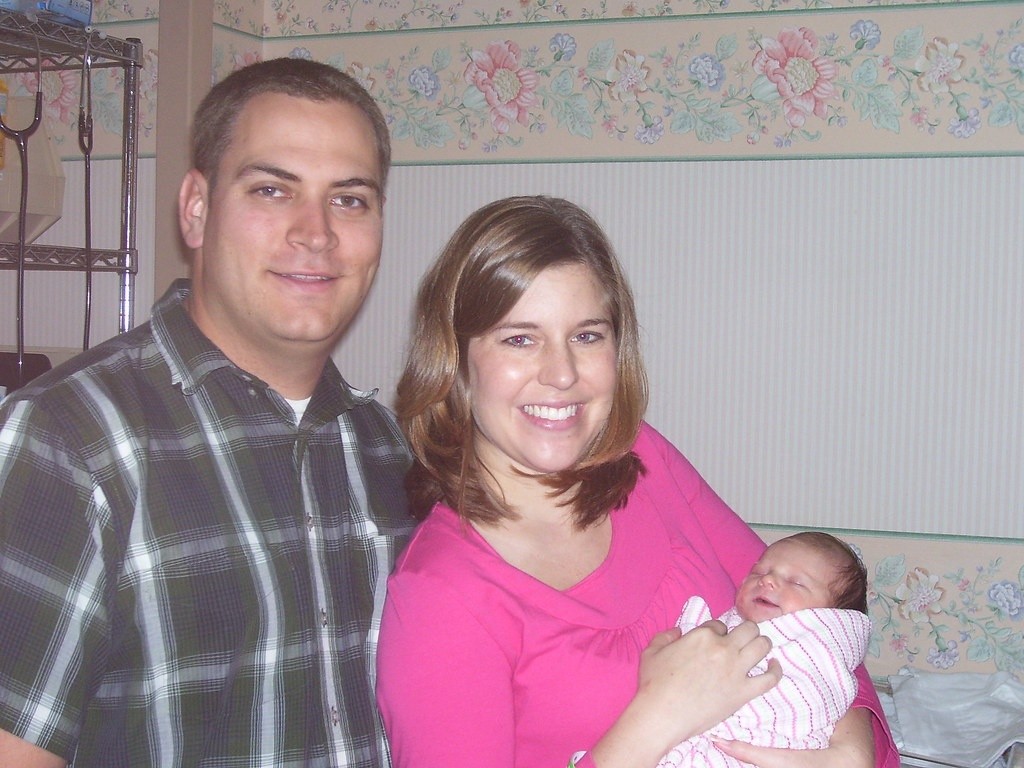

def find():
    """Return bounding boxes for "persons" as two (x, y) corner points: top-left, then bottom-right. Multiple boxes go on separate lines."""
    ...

(0, 59), (415, 768)
(655, 532), (873, 768)
(372, 191), (903, 768)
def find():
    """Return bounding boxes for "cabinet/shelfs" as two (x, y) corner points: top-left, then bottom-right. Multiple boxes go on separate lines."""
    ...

(0, 5), (144, 402)
